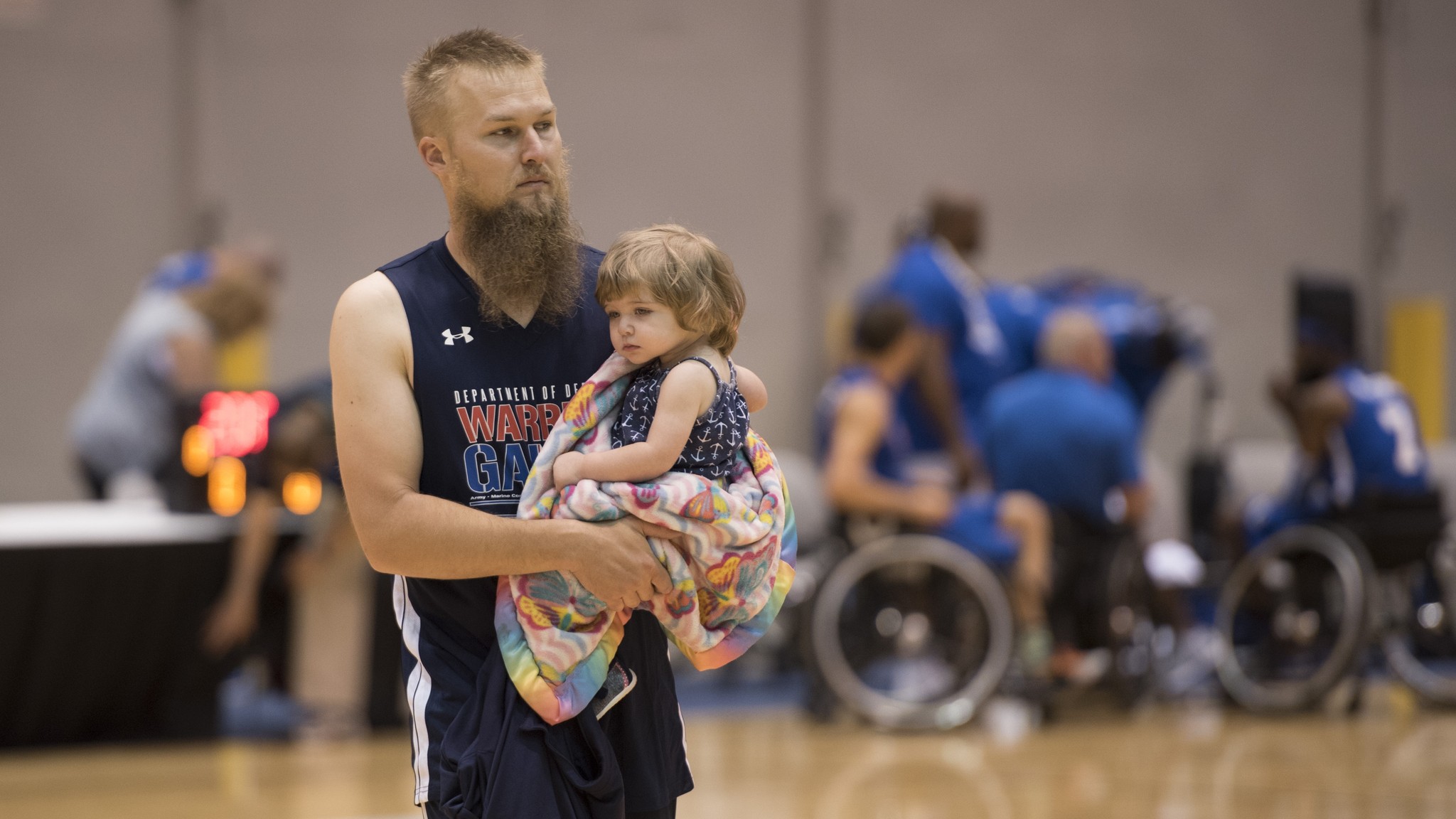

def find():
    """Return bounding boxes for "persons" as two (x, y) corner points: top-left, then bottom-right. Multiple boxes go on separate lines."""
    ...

(328, 27), (694, 819)
(549, 225), (768, 721)
(60, 227), (415, 730)
(811, 187), (1429, 691)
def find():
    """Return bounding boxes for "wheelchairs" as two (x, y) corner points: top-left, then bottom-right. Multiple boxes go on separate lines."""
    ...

(1208, 484), (1455, 720)
(777, 489), (1191, 739)
